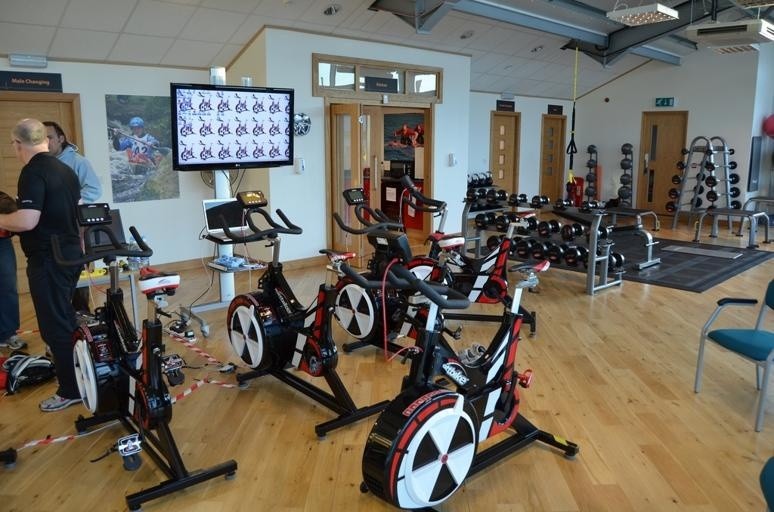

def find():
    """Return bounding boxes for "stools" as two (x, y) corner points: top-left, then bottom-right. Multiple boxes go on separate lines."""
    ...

(691, 208), (771, 249)
(604, 205), (660, 231)
(735, 196), (773, 235)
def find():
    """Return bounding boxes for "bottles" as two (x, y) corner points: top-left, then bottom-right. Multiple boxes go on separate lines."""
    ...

(128, 237), (149, 270)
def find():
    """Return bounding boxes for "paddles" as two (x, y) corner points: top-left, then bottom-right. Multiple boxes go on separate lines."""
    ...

(108, 127), (172, 158)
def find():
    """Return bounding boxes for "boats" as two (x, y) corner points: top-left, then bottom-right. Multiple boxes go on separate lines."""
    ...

(124, 147), (163, 176)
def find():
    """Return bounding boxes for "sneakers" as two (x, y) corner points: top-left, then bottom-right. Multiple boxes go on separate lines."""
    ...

(0, 335), (27, 349)
(39, 394), (83, 412)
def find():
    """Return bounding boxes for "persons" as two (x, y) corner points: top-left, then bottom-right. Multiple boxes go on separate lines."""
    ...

(0, 191), (28, 350)
(411, 123), (424, 144)
(395, 122), (418, 145)
(113, 117), (160, 165)
(43, 122), (101, 309)
(0, 119), (86, 411)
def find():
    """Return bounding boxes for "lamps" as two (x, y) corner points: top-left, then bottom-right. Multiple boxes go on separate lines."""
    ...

(710, 39), (761, 56)
(8, 53), (49, 69)
(605, 0), (679, 27)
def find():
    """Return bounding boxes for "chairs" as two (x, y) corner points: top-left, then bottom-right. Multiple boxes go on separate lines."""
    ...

(693, 278), (773, 434)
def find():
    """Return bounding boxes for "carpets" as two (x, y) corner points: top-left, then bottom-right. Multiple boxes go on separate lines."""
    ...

(469, 212), (771, 293)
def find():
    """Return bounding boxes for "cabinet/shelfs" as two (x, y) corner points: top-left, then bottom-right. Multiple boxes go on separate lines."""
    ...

(340, 171), (422, 232)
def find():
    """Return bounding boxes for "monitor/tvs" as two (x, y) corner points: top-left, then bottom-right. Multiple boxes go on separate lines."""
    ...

(202, 199), (249, 231)
(169, 82), (293, 171)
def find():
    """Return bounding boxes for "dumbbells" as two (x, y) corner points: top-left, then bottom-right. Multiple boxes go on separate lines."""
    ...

(665, 148), (708, 212)
(705, 148), (741, 216)
(467, 187), (625, 269)
